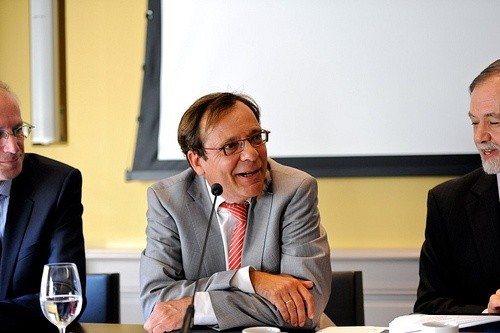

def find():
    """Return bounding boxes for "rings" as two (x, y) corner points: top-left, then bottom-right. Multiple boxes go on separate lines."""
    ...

(285, 300), (293, 303)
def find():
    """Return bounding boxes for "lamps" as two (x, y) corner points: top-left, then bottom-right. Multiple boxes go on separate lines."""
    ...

(27, 0), (65, 145)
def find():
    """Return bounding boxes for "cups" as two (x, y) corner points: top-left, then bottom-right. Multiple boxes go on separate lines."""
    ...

(241, 326), (281, 333)
(421, 320), (460, 333)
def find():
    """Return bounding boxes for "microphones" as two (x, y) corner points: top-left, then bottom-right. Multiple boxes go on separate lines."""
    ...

(179, 183), (224, 333)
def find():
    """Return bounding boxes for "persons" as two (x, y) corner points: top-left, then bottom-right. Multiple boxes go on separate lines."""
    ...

(138, 91), (332, 333)
(413, 59), (500, 315)
(0, 80), (88, 333)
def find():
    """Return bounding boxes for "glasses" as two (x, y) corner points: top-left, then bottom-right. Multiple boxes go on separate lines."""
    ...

(200, 129), (271, 156)
(0, 121), (35, 142)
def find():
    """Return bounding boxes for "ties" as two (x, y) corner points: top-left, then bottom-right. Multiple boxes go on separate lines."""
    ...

(219, 200), (250, 270)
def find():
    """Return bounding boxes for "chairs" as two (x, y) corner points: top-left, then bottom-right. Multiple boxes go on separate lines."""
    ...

(323, 271), (365, 327)
(79, 273), (119, 323)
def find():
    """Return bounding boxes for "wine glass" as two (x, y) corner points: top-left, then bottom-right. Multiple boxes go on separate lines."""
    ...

(39, 262), (83, 333)
